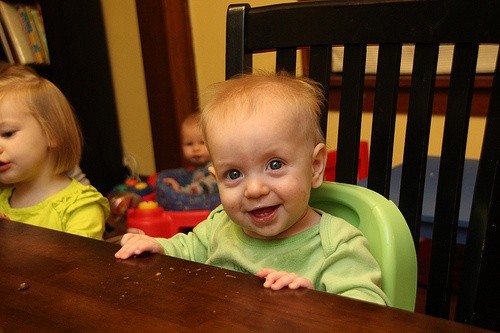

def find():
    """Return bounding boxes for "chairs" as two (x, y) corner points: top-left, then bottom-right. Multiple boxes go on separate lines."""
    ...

(155, 0), (500, 333)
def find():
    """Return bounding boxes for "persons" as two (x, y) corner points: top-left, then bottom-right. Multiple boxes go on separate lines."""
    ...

(163, 111), (218, 195)
(0, 64), (111, 241)
(114, 67), (391, 307)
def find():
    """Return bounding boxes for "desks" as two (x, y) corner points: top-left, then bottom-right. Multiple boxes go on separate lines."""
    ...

(0, 215), (484, 333)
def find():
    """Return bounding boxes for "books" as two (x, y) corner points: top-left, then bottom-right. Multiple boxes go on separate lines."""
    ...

(0, 0), (50, 65)
